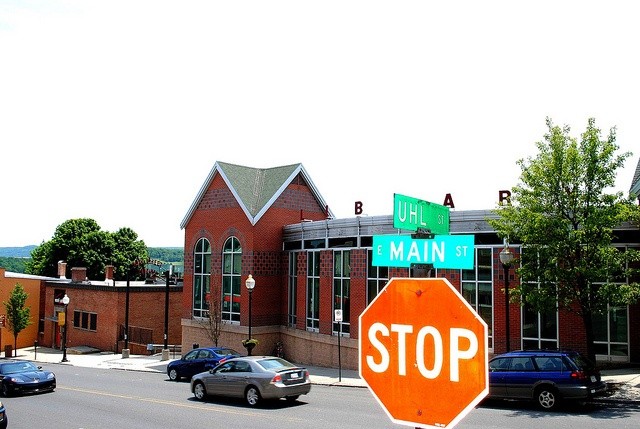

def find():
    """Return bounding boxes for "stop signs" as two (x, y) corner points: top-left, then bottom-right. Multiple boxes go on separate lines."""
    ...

(358, 277), (488, 429)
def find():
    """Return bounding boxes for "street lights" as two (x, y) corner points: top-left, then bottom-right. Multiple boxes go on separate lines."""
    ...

(59, 294), (70, 362)
(499, 246), (513, 353)
(243, 276), (255, 358)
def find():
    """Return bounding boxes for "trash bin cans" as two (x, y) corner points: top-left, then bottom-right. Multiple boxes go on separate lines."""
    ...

(5, 345), (12, 357)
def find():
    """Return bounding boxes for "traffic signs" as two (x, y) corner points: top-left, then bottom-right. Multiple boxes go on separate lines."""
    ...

(372, 234), (474, 271)
(393, 194), (449, 233)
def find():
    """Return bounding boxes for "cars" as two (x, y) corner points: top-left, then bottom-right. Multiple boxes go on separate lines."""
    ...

(167, 348), (243, 382)
(477, 349), (607, 410)
(190, 356), (312, 407)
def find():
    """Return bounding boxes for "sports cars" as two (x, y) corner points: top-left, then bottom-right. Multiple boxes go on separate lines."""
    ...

(0, 361), (55, 396)
